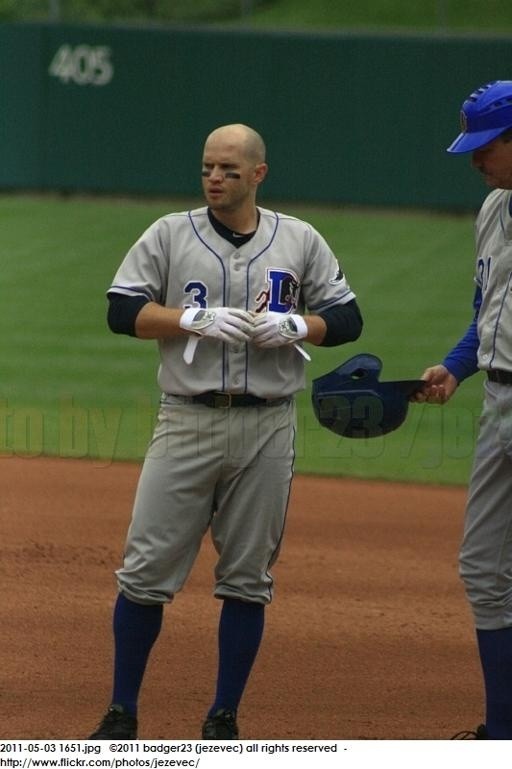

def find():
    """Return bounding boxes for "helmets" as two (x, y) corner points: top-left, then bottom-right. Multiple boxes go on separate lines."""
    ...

(447, 79), (512, 153)
(312, 354), (428, 439)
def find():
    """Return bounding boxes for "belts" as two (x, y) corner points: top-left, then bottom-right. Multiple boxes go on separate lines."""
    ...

(186, 389), (290, 409)
(485, 367), (512, 390)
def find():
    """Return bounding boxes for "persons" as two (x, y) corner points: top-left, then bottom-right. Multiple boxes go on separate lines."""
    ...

(403, 78), (510, 741)
(81, 119), (364, 739)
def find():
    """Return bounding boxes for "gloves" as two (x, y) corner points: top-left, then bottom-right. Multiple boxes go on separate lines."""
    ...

(177, 303), (253, 363)
(250, 311), (309, 362)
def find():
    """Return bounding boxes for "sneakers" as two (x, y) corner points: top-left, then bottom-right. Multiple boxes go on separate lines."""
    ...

(90, 705), (138, 741)
(201, 708), (239, 740)
